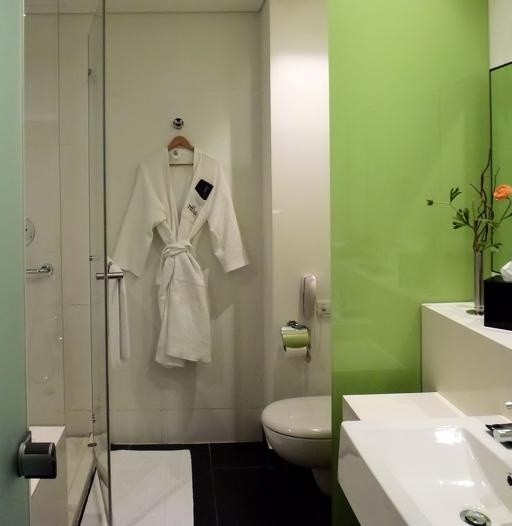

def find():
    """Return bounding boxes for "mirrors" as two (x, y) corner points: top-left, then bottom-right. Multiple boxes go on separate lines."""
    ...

(490, 62), (512, 275)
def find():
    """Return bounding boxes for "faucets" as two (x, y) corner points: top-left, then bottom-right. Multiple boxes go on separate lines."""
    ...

(491, 401), (512, 444)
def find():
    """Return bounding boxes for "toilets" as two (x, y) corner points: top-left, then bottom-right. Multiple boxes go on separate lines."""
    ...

(260, 391), (334, 495)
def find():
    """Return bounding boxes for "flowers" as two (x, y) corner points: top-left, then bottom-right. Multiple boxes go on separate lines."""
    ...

(490, 182), (512, 230)
(424, 143), (512, 314)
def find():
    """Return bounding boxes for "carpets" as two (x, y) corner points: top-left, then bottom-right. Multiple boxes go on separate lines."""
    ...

(80, 450), (195, 526)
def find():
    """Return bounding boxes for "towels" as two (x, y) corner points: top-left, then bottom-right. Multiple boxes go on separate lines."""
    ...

(105, 254), (129, 373)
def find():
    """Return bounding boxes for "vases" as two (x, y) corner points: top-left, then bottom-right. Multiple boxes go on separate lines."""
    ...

(473, 239), (490, 316)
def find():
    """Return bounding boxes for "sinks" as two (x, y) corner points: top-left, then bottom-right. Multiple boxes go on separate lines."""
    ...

(335, 414), (512, 526)
